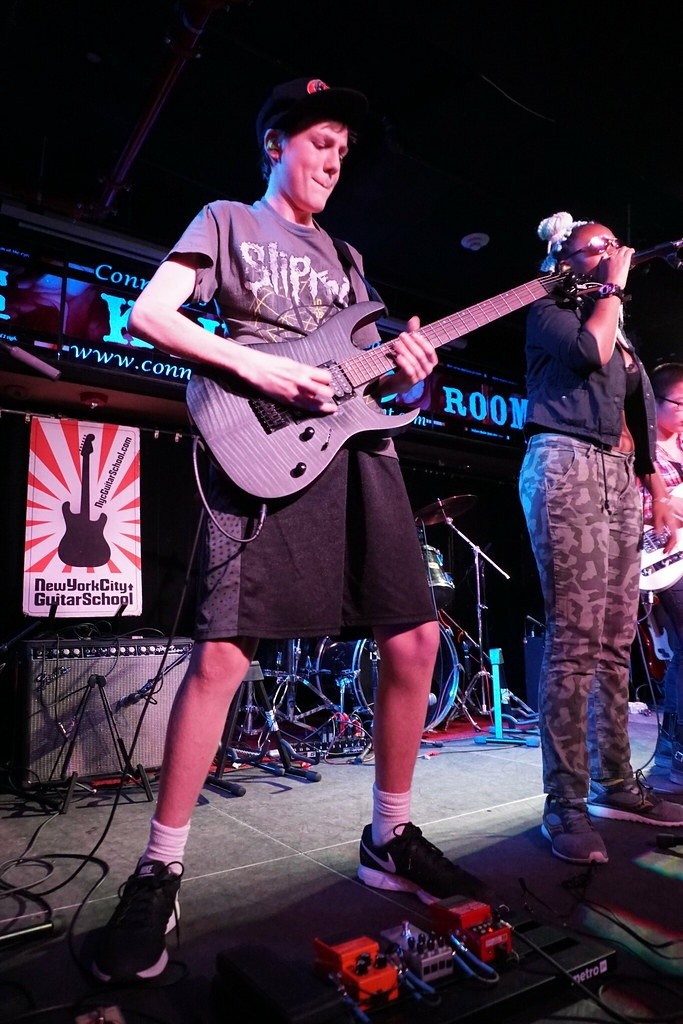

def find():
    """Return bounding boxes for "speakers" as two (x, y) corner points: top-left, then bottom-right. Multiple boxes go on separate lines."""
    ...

(524, 635), (546, 714)
(19, 636), (195, 789)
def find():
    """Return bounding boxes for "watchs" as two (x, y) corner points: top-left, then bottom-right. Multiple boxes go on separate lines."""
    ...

(597, 281), (632, 303)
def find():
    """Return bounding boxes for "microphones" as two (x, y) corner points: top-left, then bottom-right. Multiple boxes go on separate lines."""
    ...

(628, 239), (683, 271)
(428, 693), (437, 705)
(119, 692), (145, 707)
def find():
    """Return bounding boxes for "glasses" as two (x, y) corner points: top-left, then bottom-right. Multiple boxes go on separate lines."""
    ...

(655, 394), (683, 411)
(561, 236), (620, 256)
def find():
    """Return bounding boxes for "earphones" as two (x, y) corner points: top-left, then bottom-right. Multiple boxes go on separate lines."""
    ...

(561, 265), (570, 271)
(266, 140), (280, 151)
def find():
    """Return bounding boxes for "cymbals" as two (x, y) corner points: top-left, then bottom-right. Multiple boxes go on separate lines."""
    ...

(413, 494), (478, 526)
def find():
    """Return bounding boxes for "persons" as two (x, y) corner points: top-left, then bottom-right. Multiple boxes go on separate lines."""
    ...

(82, 78), (496, 986)
(638, 360), (683, 795)
(516, 211), (683, 863)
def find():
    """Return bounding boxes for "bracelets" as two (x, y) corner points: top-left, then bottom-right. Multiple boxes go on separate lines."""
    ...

(649, 497), (672, 504)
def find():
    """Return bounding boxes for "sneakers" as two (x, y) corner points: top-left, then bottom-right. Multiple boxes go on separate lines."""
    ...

(357, 820), (488, 906)
(89, 857), (186, 989)
(586, 769), (683, 826)
(540, 794), (608, 863)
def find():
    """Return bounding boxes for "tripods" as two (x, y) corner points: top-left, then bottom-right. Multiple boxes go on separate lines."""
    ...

(231, 630), (354, 759)
(443, 516), (539, 735)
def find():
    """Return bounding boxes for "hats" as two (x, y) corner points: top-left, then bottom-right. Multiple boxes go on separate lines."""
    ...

(256, 76), (368, 147)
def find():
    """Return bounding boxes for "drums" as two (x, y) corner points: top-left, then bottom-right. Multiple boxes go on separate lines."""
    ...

(315, 621), (461, 734)
(421, 543), (455, 612)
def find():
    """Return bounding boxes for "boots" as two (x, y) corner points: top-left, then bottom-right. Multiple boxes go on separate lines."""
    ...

(670, 724), (683, 785)
(654, 711), (676, 767)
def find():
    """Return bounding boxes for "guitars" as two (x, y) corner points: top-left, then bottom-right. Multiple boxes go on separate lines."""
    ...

(183, 258), (594, 507)
(638, 480), (683, 595)
